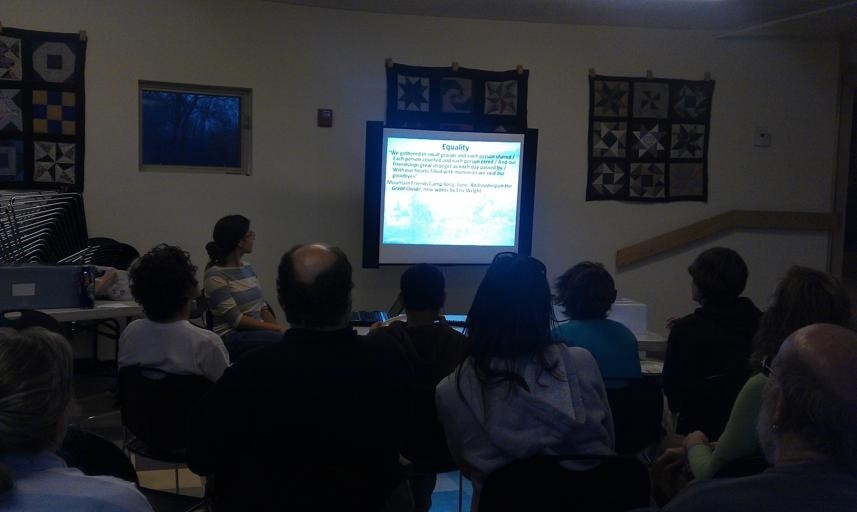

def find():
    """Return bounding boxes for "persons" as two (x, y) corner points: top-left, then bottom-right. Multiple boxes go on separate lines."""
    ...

(662, 247), (772, 416)
(201, 214), (280, 359)
(552, 261), (640, 389)
(437, 252), (616, 484)
(0, 310), (153, 512)
(187, 243), (411, 512)
(658, 322), (857, 512)
(667, 267), (850, 481)
(116, 241), (230, 458)
(369, 262), (471, 512)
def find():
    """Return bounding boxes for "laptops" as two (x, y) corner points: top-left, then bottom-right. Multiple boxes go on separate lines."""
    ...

(351, 292), (404, 327)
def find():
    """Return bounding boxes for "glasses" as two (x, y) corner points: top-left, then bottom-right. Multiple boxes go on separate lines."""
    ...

(243, 229), (256, 241)
(761, 355), (779, 381)
(489, 251), (547, 278)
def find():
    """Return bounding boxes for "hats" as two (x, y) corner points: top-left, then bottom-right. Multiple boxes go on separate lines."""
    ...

(688, 245), (749, 296)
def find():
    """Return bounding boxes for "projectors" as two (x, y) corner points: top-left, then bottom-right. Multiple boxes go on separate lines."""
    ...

(0, 266), (105, 310)
